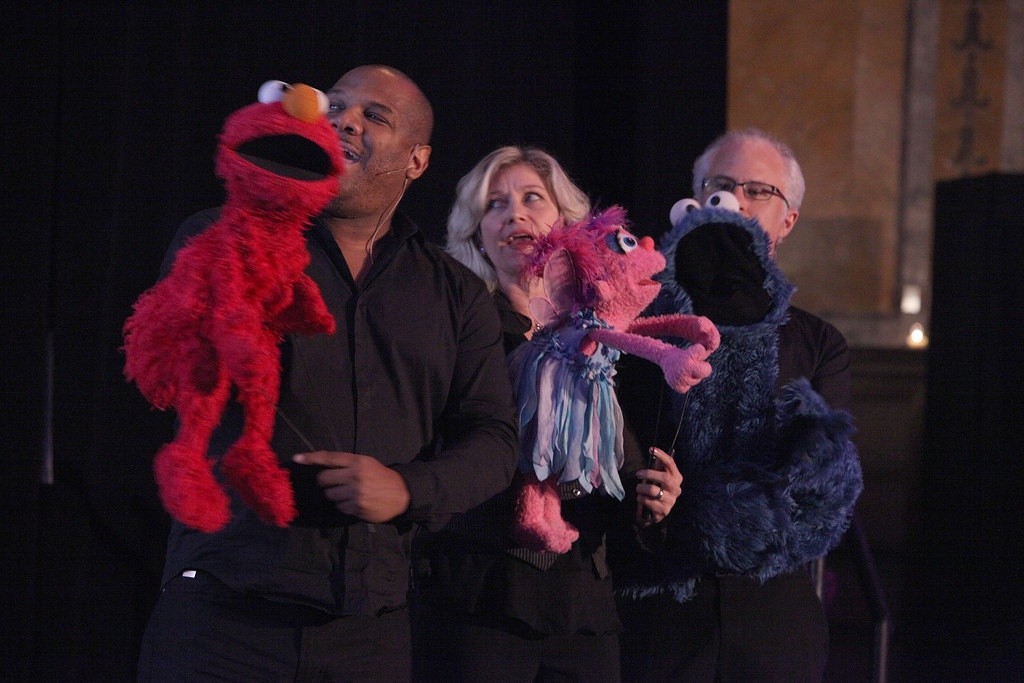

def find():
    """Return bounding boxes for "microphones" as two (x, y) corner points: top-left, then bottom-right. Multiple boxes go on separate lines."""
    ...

(480, 236), (514, 254)
(386, 143), (418, 175)
(641, 385), (690, 520)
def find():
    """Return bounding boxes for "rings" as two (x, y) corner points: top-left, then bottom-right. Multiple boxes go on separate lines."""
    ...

(654, 488), (664, 500)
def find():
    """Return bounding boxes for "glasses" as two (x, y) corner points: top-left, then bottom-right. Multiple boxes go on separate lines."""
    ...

(702, 175), (791, 209)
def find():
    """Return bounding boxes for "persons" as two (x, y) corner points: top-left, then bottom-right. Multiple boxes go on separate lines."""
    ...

(626, 123), (861, 502)
(134, 61), (526, 683)
(410, 144), (685, 683)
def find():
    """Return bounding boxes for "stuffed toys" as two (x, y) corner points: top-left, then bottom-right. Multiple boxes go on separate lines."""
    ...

(615, 189), (867, 682)
(495, 204), (721, 555)
(118, 77), (348, 536)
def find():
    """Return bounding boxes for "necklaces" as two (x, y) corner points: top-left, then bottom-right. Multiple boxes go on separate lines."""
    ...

(533, 321), (543, 330)
